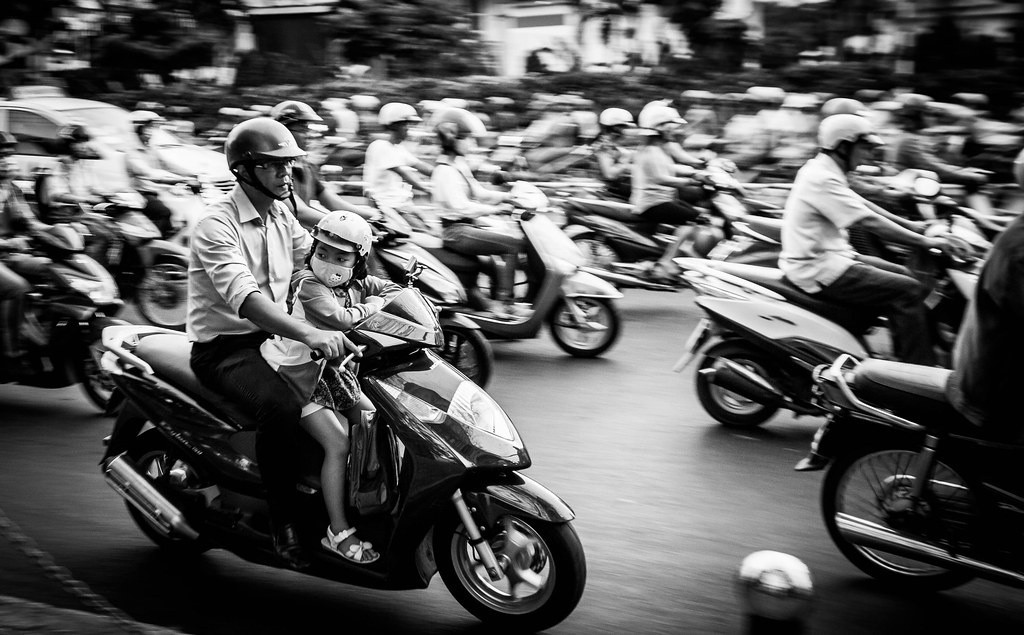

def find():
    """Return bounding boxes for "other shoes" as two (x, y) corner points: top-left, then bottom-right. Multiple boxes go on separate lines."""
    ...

(498, 302), (534, 322)
(653, 259), (684, 282)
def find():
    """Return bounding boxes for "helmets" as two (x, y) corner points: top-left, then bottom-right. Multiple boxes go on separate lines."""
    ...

(224, 117), (308, 169)
(818, 113), (887, 152)
(0, 68), (932, 173)
(312, 210), (372, 260)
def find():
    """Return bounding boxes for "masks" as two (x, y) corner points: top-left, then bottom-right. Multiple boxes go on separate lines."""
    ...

(311, 253), (356, 288)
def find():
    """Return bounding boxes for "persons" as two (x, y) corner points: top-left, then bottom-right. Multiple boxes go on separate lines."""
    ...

(941, 148), (1024, 465)
(185, 117), (401, 565)
(0, 84), (1024, 368)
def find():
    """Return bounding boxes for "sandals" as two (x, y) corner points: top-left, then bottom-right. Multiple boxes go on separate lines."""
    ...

(321, 525), (380, 565)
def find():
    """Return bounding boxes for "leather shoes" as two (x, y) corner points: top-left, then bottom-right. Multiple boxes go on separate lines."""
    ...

(271, 523), (307, 570)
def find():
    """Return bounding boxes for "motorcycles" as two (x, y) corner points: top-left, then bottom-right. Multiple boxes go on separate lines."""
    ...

(1, 93), (1024, 433)
(794, 351), (1024, 602)
(92, 252), (590, 635)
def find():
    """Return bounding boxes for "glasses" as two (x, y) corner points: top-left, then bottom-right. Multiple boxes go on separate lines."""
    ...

(255, 156), (298, 171)
